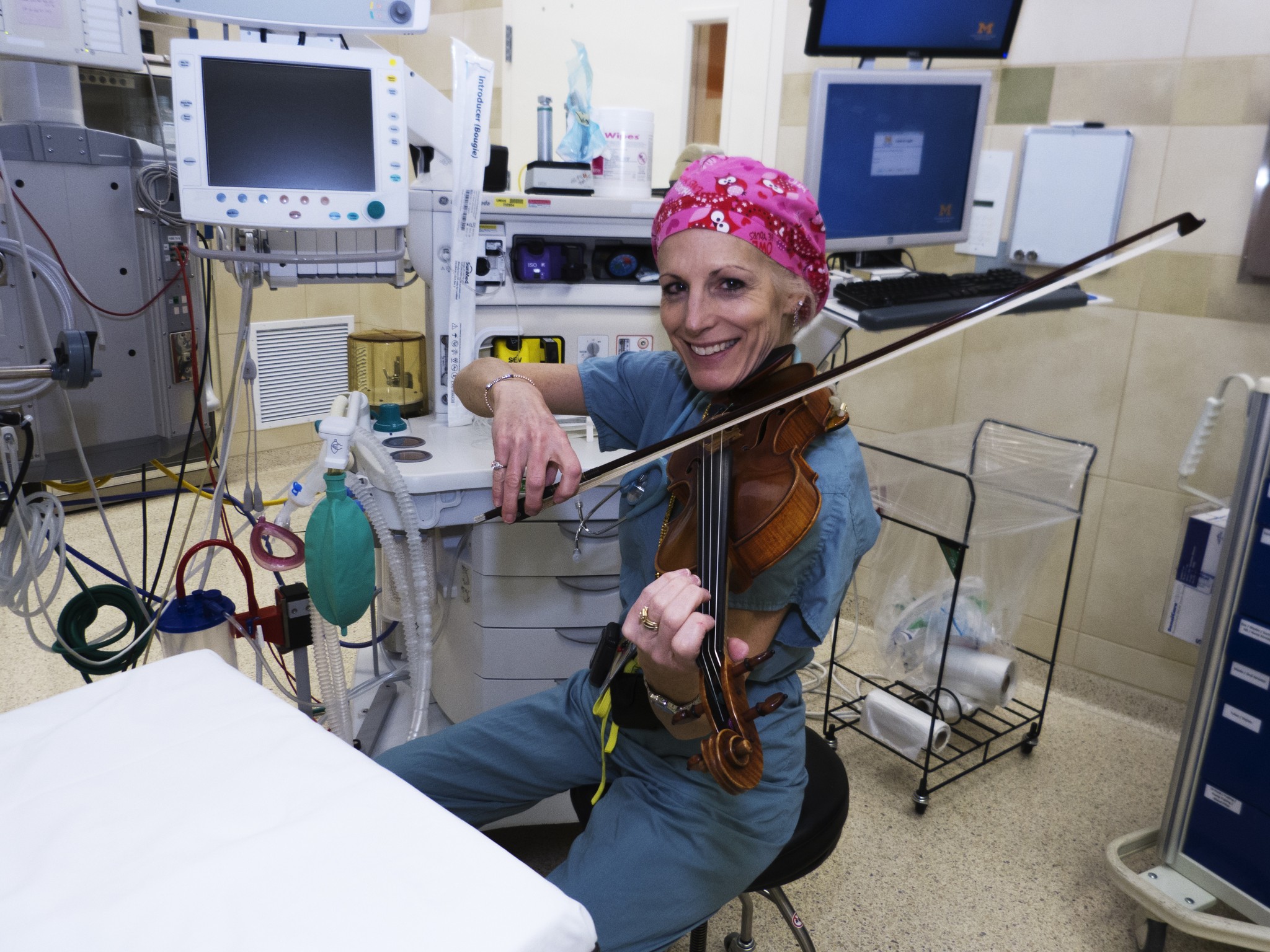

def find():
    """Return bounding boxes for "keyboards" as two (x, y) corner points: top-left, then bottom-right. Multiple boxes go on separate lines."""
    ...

(833, 267), (1087, 331)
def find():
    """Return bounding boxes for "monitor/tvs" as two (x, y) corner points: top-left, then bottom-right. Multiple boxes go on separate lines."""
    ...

(169, 38), (410, 230)
(804, 0), (1022, 59)
(804, 70), (993, 278)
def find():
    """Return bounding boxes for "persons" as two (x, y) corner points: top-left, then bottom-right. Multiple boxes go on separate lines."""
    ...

(370, 153), (880, 952)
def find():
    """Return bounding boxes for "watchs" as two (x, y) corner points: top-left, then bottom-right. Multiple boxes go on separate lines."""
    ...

(644, 675), (702, 715)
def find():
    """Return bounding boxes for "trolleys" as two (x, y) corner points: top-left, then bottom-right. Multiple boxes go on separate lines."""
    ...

(821, 418), (1098, 814)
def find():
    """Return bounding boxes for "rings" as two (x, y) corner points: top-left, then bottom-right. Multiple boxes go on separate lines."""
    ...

(491, 461), (507, 471)
(638, 606), (659, 632)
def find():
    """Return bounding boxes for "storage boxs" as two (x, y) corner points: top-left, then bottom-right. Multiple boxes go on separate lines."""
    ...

(525, 160), (592, 194)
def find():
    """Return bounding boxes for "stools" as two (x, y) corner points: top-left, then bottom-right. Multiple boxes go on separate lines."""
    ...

(571, 724), (850, 952)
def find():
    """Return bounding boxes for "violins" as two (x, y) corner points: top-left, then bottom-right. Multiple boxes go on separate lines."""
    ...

(654, 361), (835, 797)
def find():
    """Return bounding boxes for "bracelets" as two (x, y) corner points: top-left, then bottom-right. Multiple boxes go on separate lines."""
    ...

(484, 375), (536, 414)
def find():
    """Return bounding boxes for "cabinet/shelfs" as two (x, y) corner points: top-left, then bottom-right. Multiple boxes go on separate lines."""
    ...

(354, 413), (637, 724)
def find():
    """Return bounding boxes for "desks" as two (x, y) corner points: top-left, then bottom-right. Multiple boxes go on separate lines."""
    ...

(0, 648), (596, 952)
